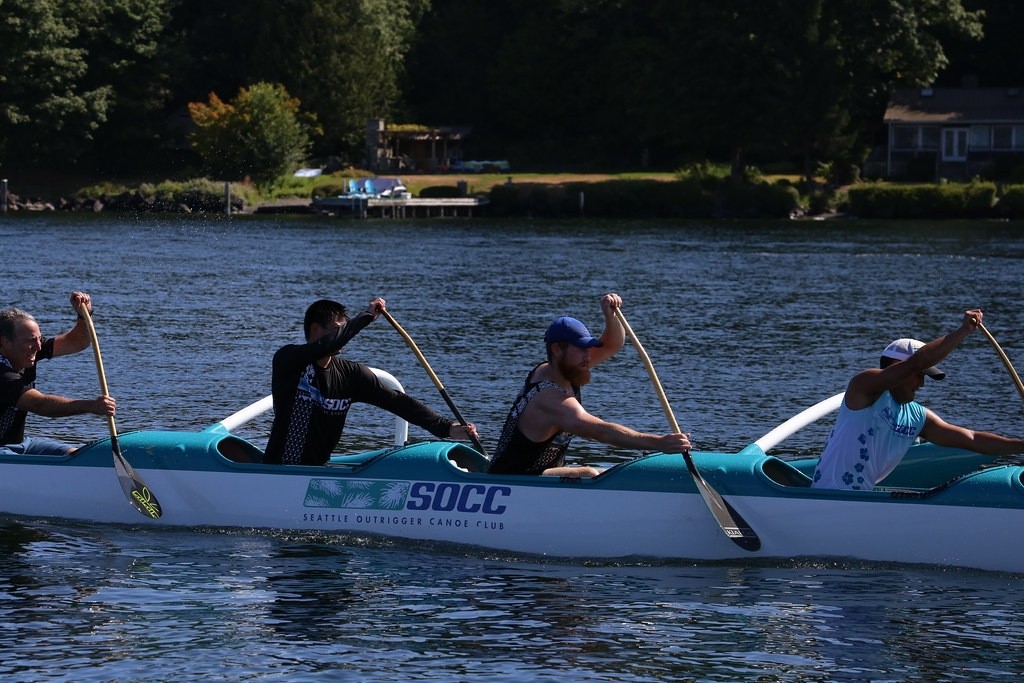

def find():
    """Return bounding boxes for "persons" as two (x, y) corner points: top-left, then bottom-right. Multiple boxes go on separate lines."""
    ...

(486, 291), (692, 478)
(0, 290), (115, 456)
(811, 308), (1024, 492)
(261, 297), (480, 467)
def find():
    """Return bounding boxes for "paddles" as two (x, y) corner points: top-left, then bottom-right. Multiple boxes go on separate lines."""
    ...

(608, 303), (764, 555)
(378, 307), (489, 455)
(81, 301), (163, 519)
(972, 317), (1024, 399)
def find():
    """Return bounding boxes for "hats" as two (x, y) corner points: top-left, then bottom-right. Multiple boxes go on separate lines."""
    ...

(545, 317), (604, 348)
(881, 338), (946, 381)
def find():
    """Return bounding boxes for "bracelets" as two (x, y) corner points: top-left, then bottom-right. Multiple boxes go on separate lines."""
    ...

(77, 310), (94, 320)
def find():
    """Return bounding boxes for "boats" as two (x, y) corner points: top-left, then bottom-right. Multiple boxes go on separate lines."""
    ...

(0, 430), (1024, 573)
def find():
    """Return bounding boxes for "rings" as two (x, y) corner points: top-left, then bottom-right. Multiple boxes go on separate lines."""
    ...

(375, 299), (376, 301)
